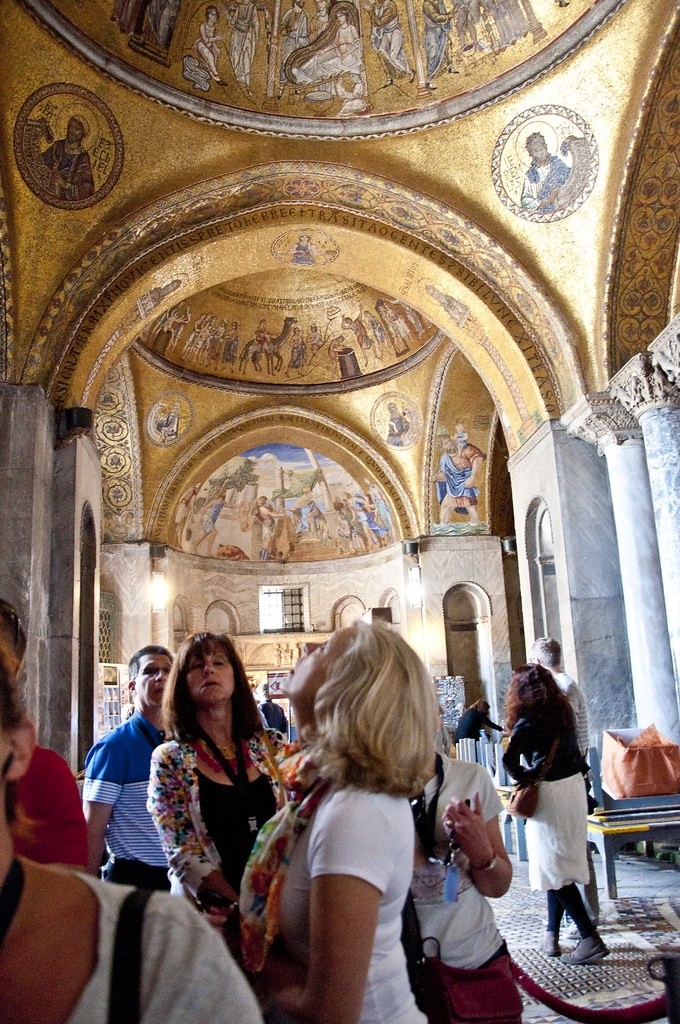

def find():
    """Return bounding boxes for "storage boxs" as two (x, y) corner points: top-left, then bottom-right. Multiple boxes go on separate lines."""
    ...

(601, 728), (680, 800)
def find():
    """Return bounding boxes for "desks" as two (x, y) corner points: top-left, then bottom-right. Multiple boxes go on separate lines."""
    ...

(586, 821), (680, 899)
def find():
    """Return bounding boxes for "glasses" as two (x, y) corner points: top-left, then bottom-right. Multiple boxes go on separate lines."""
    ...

(0, 598), (20, 651)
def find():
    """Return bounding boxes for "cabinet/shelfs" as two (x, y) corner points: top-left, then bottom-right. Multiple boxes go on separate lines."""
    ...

(97, 663), (129, 739)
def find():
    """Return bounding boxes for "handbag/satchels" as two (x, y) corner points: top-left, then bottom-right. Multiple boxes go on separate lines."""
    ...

(506, 784), (538, 818)
(423, 955), (524, 1024)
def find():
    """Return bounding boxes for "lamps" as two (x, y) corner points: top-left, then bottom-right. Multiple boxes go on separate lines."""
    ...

(402, 543), (418, 562)
(503, 539), (517, 557)
(150, 542), (167, 560)
(56, 407), (92, 450)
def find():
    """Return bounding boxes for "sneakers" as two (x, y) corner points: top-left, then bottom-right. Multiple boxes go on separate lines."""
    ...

(561, 935), (610, 964)
(543, 931), (561, 957)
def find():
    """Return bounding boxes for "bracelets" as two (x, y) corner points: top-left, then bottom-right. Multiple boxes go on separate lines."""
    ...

(479, 853), (499, 871)
(229, 902), (238, 909)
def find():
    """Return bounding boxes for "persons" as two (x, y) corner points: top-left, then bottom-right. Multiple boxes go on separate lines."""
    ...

(239, 617), (441, 1024)
(451, 699), (506, 748)
(0, 597), (524, 1024)
(504, 636), (614, 966)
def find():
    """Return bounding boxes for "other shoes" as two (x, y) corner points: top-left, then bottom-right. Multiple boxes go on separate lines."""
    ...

(564, 923), (580, 939)
